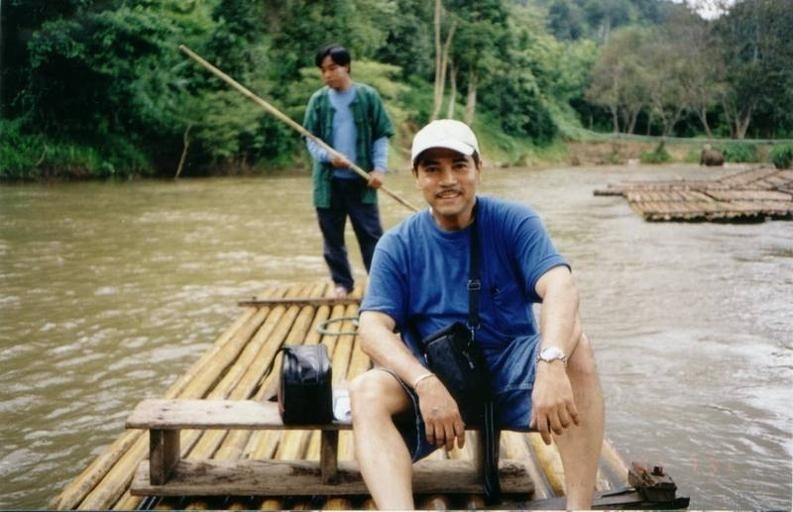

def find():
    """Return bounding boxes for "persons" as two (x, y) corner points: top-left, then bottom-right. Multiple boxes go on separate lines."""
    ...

(302, 44), (395, 293)
(349, 117), (604, 510)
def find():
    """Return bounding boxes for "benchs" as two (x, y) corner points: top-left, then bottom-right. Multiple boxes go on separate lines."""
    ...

(127, 384), (538, 506)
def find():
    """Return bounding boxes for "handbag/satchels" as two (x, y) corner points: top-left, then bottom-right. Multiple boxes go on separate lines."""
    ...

(419, 322), (491, 400)
(280, 344), (332, 426)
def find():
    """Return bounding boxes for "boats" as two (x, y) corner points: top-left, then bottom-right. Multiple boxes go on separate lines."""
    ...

(593, 161), (793, 223)
(29, 269), (695, 510)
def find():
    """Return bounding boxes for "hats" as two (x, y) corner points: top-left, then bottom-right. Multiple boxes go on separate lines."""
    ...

(411, 119), (479, 172)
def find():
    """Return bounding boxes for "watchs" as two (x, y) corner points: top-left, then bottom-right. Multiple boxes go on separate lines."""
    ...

(535, 345), (570, 364)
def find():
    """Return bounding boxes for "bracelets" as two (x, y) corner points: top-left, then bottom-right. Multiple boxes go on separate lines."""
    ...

(409, 371), (433, 389)
